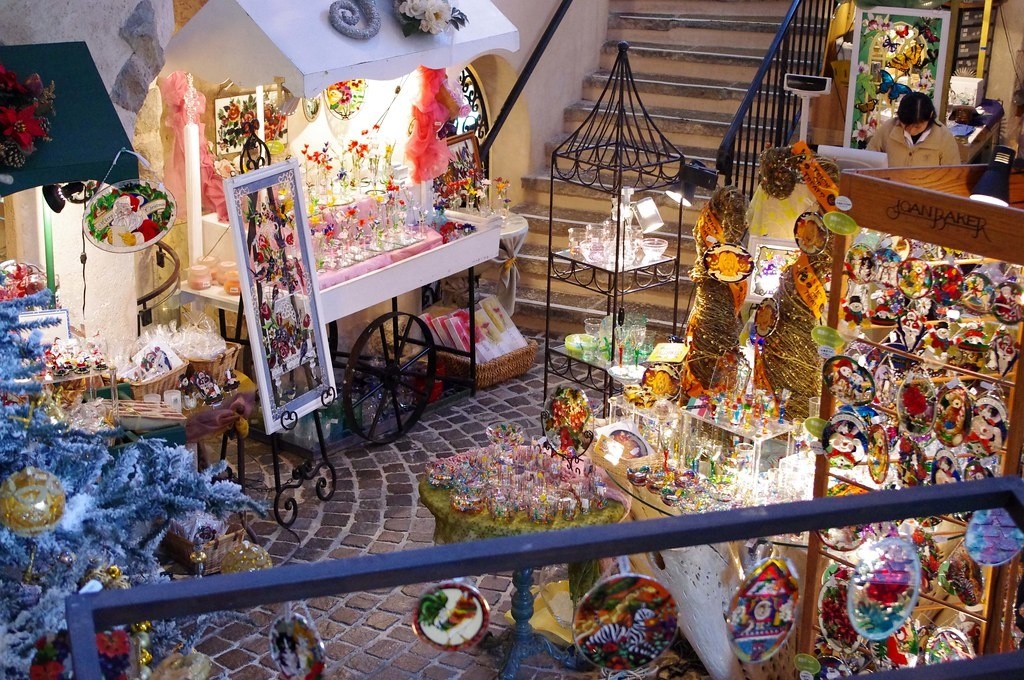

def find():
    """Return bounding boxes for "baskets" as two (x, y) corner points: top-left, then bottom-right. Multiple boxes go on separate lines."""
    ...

(102, 351), (189, 402)
(177, 341), (241, 384)
(399, 319), (537, 390)
(155, 518), (245, 576)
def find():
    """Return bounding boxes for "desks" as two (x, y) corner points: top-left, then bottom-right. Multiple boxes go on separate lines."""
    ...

(945, 99), (1005, 164)
(446, 207), (529, 318)
(418, 447), (633, 680)
(181, 370), (254, 493)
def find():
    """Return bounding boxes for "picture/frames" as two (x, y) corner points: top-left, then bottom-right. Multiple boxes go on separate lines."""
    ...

(222, 158), (338, 435)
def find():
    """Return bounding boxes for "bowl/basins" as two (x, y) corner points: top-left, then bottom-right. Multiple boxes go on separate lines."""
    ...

(629, 458), (700, 507)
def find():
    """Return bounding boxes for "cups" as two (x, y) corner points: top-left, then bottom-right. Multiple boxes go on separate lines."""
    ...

(809, 397), (821, 418)
(164, 390), (182, 415)
(144, 393), (162, 402)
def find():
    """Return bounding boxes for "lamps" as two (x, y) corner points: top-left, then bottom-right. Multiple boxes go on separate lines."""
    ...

(968, 144), (1016, 208)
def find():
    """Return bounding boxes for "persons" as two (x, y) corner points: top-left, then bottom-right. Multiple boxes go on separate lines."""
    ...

(866, 93), (960, 167)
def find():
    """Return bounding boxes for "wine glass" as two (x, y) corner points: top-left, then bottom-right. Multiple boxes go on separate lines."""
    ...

(790, 416), (807, 461)
(662, 414), (752, 476)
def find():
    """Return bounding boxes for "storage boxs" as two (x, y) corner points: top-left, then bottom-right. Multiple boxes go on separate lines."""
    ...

(946, 75), (986, 108)
(417, 296), (530, 368)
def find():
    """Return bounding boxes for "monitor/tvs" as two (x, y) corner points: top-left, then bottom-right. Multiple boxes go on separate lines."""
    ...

(816, 144), (889, 179)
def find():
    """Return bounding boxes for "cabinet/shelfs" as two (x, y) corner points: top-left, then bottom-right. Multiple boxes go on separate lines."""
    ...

(580, 391), (808, 675)
(545, 40), (686, 420)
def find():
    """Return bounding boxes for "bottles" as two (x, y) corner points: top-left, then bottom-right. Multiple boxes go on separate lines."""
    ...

(682, 461), (815, 544)
(428, 424), (608, 527)
(188, 255), (240, 296)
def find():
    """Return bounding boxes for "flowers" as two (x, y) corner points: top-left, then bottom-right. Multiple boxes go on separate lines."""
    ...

(392, 0), (469, 37)
(0, 64), (56, 169)
